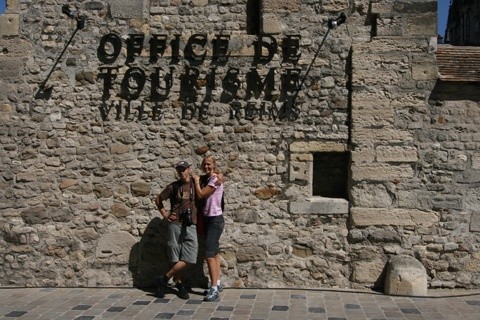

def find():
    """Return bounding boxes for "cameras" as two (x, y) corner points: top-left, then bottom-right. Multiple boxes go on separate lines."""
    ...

(182, 208), (192, 226)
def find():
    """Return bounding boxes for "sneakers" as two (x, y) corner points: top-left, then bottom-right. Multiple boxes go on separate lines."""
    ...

(152, 276), (165, 298)
(204, 287), (219, 302)
(178, 287), (189, 299)
(206, 283), (223, 292)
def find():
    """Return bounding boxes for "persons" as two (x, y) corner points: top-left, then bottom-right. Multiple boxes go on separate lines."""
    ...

(193, 155), (225, 302)
(155, 161), (224, 299)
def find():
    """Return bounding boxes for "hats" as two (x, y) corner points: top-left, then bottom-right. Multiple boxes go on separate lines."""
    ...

(174, 161), (189, 169)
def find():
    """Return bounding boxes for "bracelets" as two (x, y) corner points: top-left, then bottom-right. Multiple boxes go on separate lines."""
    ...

(194, 183), (200, 186)
